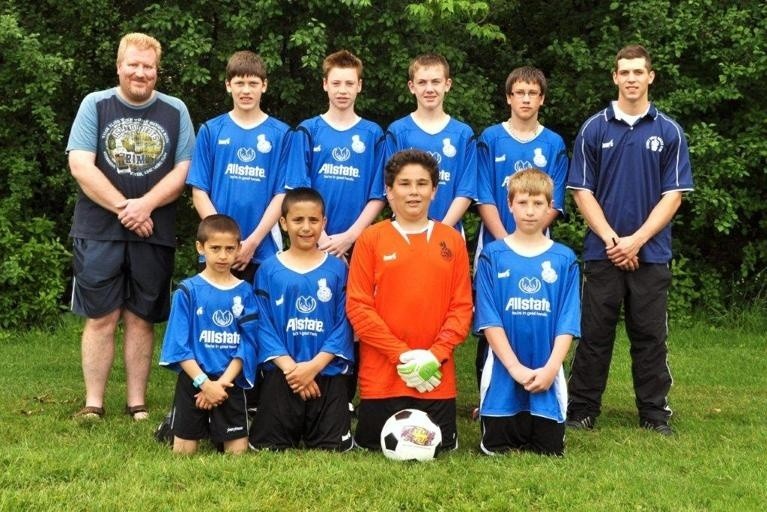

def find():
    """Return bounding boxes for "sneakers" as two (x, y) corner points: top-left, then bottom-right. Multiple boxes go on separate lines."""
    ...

(125, 405), (150, 423)
(154, 412), (172, 442)
(77, 407), (107, 423)
(647, 425), (679, 439)
(565, 411), (596, 431)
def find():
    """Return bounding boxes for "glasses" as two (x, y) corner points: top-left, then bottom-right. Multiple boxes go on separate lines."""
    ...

(509, 90), (544, 98)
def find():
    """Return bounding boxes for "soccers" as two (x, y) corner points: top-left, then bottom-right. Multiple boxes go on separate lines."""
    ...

(380, 409), (443, 463)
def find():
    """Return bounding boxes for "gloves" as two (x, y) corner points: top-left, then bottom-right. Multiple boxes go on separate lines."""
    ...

(396, 350), (442, 393)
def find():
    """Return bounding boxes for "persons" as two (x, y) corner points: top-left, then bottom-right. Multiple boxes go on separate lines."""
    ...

(283, 50), (386, 270)
(473, 67), (567, 416)
(344, 151), (470, 451)
(64, 33), (195, 422)
(472, 169), (582, 455)
(385, 54), (477, 253)
(247, 187), (358, 452)
(185, 52), (293, 286)
(566, 46), (694, 440)
(153, 214), (257, 454)
